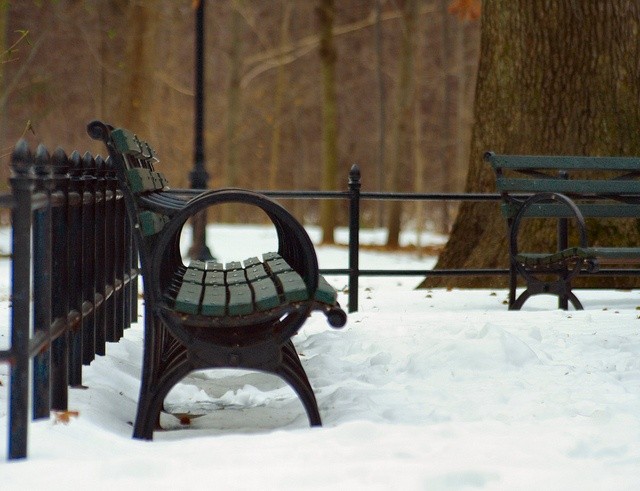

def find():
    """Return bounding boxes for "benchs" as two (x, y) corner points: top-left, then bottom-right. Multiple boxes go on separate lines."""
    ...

(482, 151), (639, 311)
(87, 119), (347, 441)
